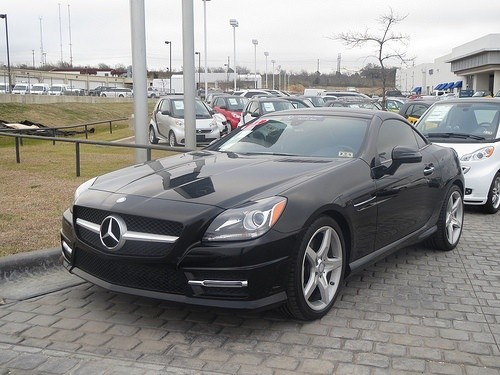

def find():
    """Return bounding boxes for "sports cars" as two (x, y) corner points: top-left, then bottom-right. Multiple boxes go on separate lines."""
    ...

(58, 105), (466, 323)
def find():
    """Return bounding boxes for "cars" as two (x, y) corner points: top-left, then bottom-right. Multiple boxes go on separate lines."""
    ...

(0, 82), (160, 99)
(197, 85), (477, 138)
(414, 96), (500, 214)
(148, 94), (221, 151)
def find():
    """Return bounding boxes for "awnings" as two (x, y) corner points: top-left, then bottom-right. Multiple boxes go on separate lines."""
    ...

(439, 83), (448, 90)
(445, 82), (454, 89)
(412, 87), (421, 92)
(437, 84), (443, 89)
(434, 84), (440, 90)
(452, 81), (462, 88)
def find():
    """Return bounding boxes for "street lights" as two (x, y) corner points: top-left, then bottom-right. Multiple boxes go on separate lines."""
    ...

(164, 41), (171, 94)
(264, 52), (269, 89)
(271, 59), (290, 91)
(195, 51), (201, 96)
(251, 38), (258, 89)
(0, 13), (12, 94)
(229, 18), (239, 91)
